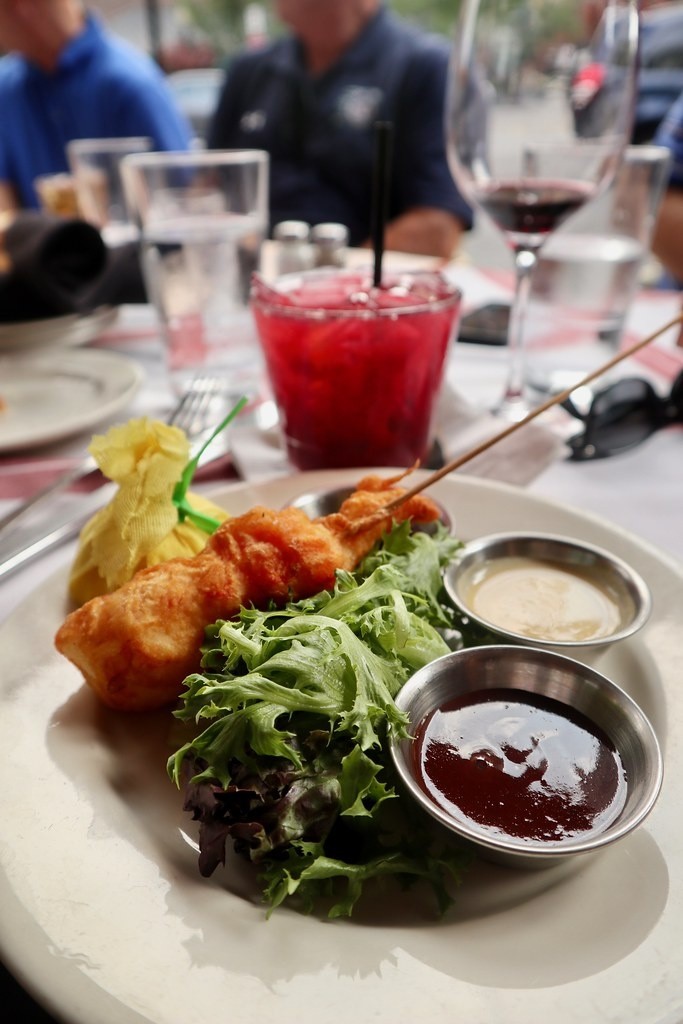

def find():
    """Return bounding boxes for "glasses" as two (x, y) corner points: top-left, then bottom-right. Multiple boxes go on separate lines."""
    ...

(561, 367), (683, 461)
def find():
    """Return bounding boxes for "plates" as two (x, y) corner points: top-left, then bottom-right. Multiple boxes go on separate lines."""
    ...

(0, 469), (683, 1023)
(0, 343), (145, 451)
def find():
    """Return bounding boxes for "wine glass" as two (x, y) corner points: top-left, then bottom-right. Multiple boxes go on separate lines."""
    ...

(445, 0), (639, 431)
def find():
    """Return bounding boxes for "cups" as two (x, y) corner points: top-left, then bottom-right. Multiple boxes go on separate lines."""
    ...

(247, 264), (461, 471)
(120, 149), (268, 393)
(35, 138), (150, 249)
(521, 145), (672, 399)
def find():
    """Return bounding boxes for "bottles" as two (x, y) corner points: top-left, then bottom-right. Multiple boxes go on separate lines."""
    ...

(271, 219), (352, 274)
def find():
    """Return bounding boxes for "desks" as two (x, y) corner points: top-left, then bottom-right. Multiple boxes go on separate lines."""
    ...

(0, 244), (683, 1024)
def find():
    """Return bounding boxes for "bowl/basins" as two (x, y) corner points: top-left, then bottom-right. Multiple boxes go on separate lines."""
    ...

(386, 645), (665, 871)
(442, 530), (654, 667)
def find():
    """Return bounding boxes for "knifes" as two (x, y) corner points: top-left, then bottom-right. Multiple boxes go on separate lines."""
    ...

(0, 399), (282, 582)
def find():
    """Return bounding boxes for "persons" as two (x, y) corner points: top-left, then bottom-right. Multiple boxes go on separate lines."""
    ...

(622, 85), (683, 292)
(208, 1), (483, 289)
(0, 0), (202, 217)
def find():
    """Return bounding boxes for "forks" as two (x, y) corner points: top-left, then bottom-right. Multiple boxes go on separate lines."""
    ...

(0, 377), (219, 534)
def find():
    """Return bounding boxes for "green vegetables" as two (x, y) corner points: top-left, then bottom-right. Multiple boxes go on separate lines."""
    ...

(166, 517), (500, 926)
